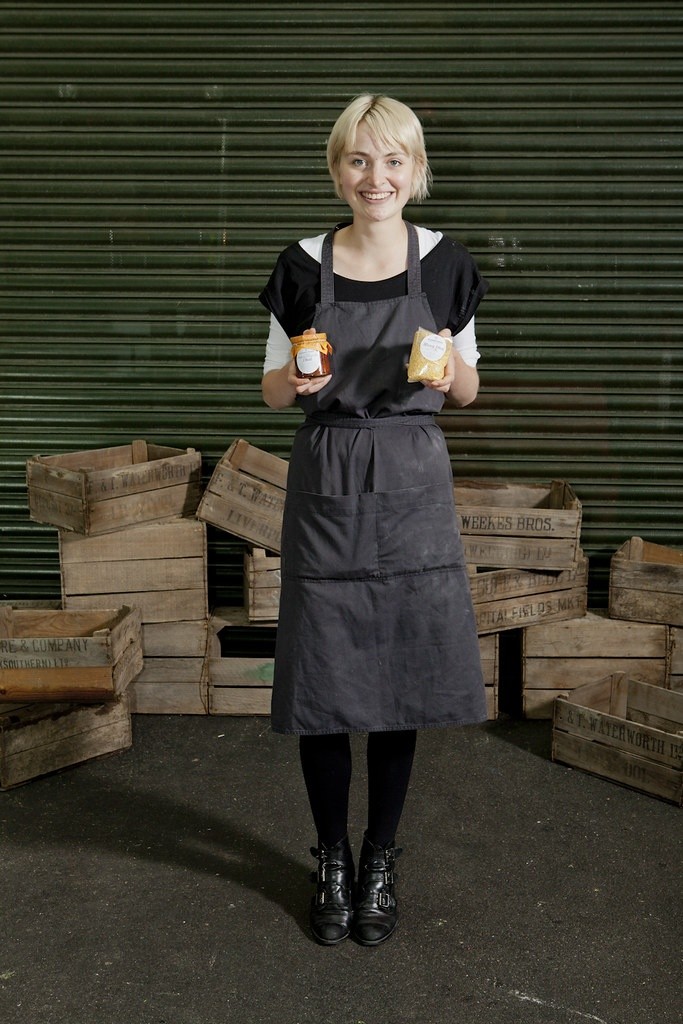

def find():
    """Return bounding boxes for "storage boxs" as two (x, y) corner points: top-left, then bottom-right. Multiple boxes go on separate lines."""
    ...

(2, 437), (683, 806)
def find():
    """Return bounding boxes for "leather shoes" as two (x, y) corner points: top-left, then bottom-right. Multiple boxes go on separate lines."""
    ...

(316, 836), (401, 945)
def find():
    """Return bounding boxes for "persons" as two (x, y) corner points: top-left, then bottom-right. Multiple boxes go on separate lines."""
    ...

(254, 91), (489, 947)
